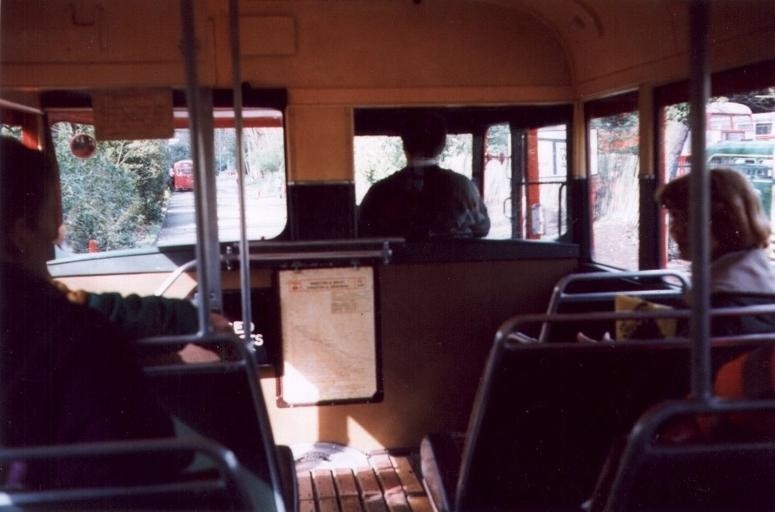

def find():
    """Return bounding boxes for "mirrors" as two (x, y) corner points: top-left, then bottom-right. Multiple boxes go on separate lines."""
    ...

(69, 132), (98, 161)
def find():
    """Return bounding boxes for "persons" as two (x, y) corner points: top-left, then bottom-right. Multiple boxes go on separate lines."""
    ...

(0, 133), (259, 509)
(575, 168), (774, 347)
(358, 116), (492, 242)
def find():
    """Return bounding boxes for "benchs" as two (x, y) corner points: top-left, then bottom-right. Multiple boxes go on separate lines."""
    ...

(415, 303), (775, 512)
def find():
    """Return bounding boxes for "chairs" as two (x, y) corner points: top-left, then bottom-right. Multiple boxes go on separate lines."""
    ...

(126, 329), (301, 512)
(0, 434), (257, 512)
(538, 268), (689, 342)
(583, 397), (775, 511)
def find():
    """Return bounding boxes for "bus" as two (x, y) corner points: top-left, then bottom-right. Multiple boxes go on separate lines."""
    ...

(172, 159), (200, 195)
(704, 138), (774, 237)
